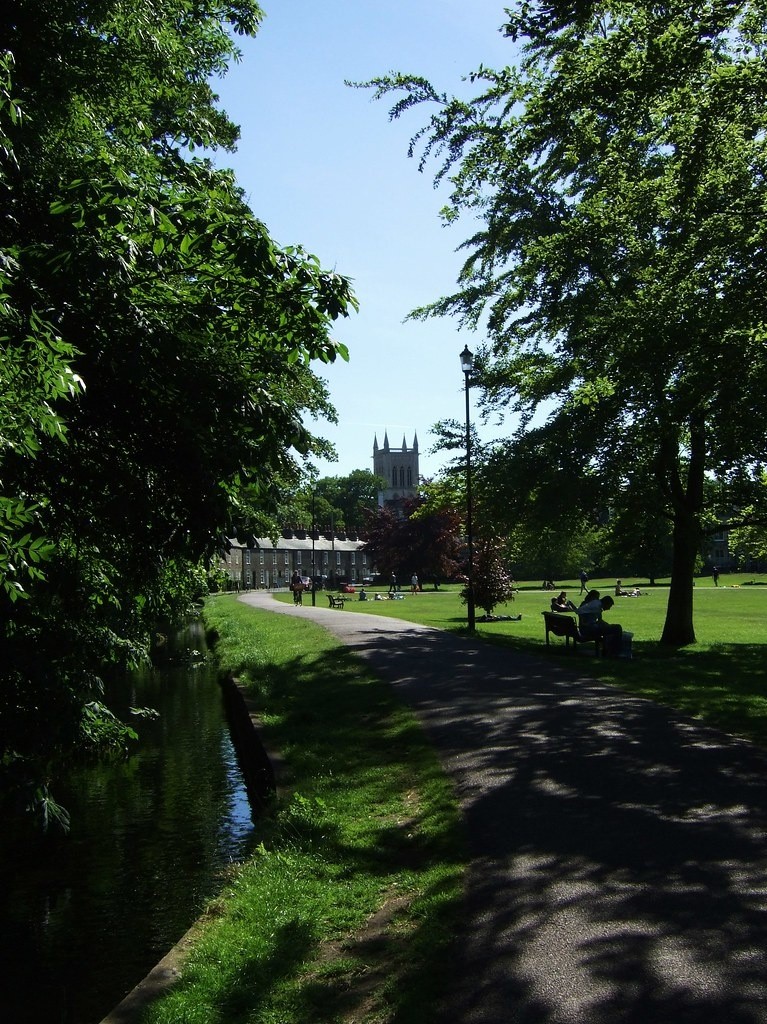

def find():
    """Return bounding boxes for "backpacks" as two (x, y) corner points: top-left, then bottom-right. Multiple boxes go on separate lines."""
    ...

(583, 574), (588, 581)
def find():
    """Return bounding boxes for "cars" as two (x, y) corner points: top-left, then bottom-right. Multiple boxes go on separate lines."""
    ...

(290, 576), (313, 591)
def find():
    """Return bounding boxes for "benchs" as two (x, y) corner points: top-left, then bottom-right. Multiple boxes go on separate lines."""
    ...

(326, 594), (343, 609)
(541, 611), (600, 656)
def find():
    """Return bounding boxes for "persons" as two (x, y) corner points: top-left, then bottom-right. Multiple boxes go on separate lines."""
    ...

(575, 590), (608, 624)
(712, 566), (719, 587)
(388, 571), (396, 592)
(550, 591), (578, 612)
(542, 581), (555, 590)
(615, 580), (628, 596)
(411, 572), (418, 595)
(577, 595), (622, 658)
(359, 589), (366, 600)
(578, 568), (590, 596)
(290, 570), (303, 604)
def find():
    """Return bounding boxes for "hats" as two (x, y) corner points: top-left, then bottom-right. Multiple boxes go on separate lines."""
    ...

(552, 598), (558, 601)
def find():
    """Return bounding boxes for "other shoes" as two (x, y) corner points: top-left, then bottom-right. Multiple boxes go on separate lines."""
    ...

(608, 651), (627, 659)
(412, 594), (417, 595)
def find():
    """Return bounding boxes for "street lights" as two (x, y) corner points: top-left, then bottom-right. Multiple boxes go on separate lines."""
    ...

(458, 342), (476, 630)
(309, 479), (318, 606)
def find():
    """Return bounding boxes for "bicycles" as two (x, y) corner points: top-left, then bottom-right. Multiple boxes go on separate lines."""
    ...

(293, 585), (304, 607)
